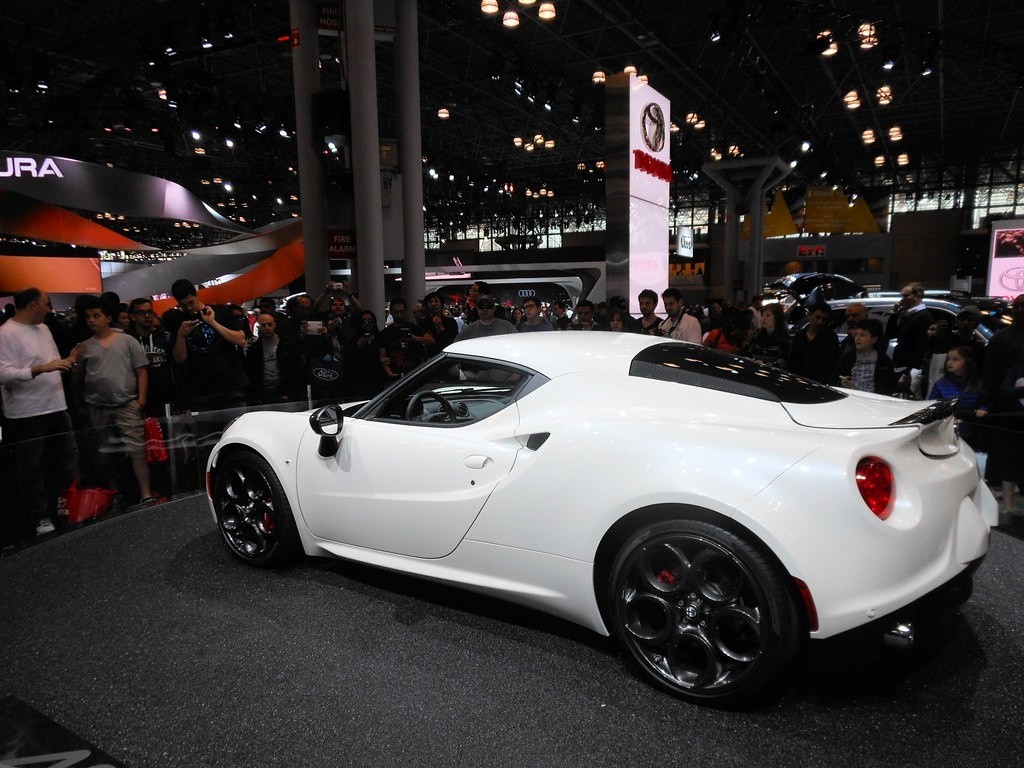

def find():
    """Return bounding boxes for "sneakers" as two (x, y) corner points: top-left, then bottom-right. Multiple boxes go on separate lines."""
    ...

(35, 519), (56, 536)
(56, 497), (68, 516)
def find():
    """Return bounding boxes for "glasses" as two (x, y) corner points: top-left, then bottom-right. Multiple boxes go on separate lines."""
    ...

(524, 304), (537, 310)
(360, 318), (375, 325)
(478, 304), (493, 309)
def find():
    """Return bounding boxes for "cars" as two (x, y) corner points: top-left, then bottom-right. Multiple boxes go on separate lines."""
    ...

(782, 289), (1024, 398)
(761, 271), (871, 302)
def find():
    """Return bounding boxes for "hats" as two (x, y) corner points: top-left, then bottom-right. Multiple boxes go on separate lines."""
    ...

(957, 305), (981, 321)
(478, 294), (495, 306)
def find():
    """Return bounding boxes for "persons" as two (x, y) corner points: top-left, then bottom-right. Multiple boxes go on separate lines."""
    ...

(884, 282), (1024, 528)
(0, 279), (472, 534)
(453, 282), (664, 341)
(648, 288), (899, 396)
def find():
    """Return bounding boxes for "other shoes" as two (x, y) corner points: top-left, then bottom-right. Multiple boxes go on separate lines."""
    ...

(998, 505), (1016, 528)
(991, 484), (1019, 497)
(108, 495), (119, 514)
(139, 494), (156, 504)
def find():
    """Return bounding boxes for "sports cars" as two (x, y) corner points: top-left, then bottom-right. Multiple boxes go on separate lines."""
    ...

(205, 331), (1004, 704)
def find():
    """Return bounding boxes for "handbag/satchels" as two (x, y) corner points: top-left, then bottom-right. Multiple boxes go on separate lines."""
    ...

(66, 477), (120, 523)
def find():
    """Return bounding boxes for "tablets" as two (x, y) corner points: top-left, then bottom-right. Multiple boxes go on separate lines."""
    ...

(302, 321), (323, 335)
